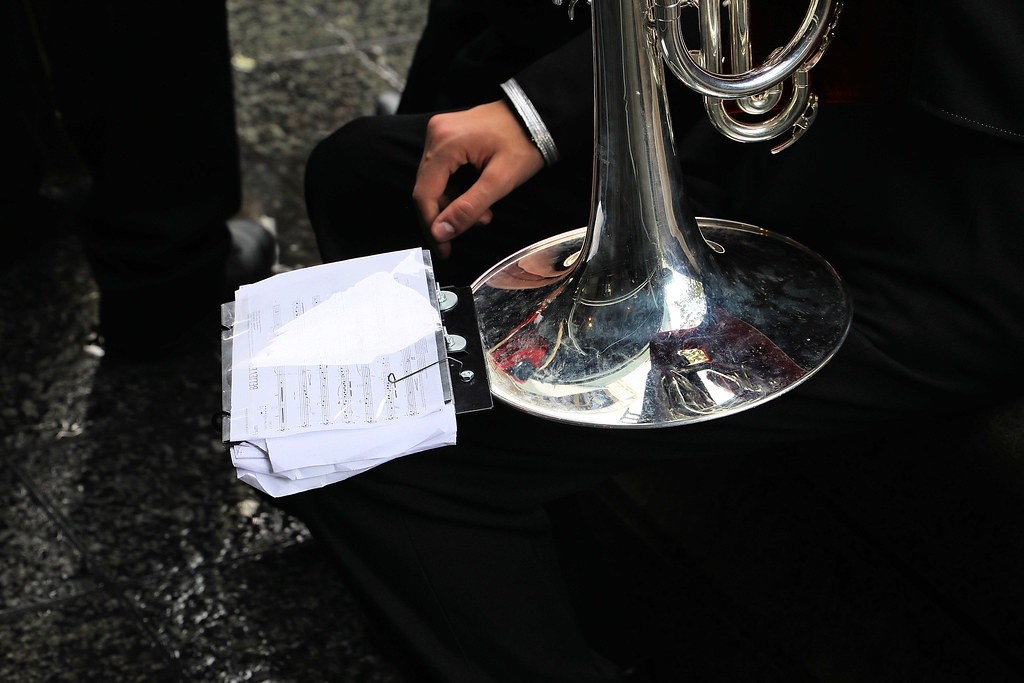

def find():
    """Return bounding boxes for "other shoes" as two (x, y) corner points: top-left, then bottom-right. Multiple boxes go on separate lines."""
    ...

(104, 224), (276, 369)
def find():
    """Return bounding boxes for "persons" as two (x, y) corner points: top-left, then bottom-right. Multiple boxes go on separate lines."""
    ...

(0, 0), (1024, 683)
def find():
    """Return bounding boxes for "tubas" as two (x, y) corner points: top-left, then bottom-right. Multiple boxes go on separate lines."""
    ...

(470, 1), (854, 429)
